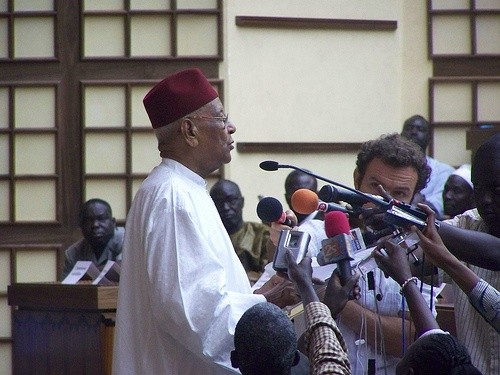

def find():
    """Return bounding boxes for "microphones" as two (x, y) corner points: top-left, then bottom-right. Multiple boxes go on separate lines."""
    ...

(259, 161), (429, 220)
(322, 211), (356, 300)
(290, 189), (354, 216)
(257, 197), (294, 228)
(318, 184), (388, 205)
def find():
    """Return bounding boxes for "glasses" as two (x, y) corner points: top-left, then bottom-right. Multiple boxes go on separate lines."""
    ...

(186, 114), (229, 124)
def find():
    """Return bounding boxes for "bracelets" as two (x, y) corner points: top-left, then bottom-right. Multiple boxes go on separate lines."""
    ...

(400, 277), (418, 297)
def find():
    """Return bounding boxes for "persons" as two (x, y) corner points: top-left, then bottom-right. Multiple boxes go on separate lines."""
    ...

(62, 198), (124, 282)
(208, 115), (500, 375)
(112, 68), (326, 375)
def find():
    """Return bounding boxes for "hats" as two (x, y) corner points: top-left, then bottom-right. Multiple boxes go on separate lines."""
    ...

(142, 68), (218, 129)
